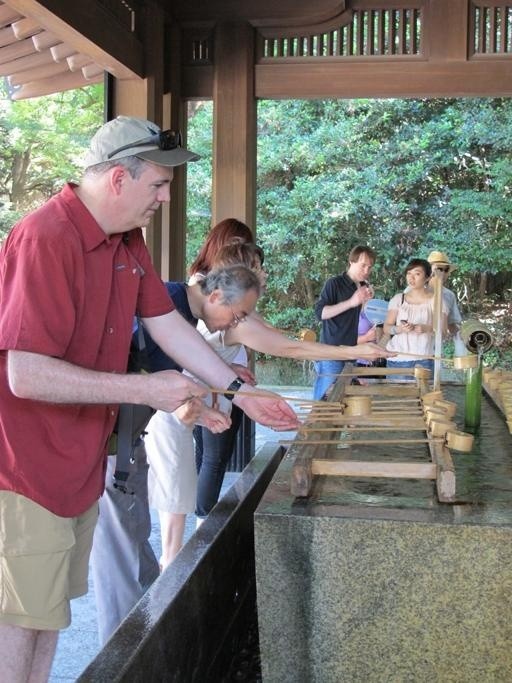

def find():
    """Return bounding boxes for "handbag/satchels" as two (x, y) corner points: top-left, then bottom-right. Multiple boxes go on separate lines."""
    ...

(376, 324), (393, 348)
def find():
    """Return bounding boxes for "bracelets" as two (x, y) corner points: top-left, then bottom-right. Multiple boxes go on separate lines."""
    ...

(224, 376), (245, 401)
(391, 326), (398, 335)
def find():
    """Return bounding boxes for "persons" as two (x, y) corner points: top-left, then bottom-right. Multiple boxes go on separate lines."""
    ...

(382, 257), (449, 385)
(142, 240), (398, 574)
(357, 280), (378, 379)
(313, 245), (378, 402)
(186, 217), (277, 531)
(90, 263), (261, 650)
(403, 251), (462, 380)
(0, 115), (302, 683)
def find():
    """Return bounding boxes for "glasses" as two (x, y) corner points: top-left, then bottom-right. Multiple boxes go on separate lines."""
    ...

(438, 268), (448, 272)
(233, 312), (246, 324)
(108, 129), (182, 158)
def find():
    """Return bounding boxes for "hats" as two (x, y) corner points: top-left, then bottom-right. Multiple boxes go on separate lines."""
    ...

(428, 251), (456, 272)
(85, 116), (200, 170)
